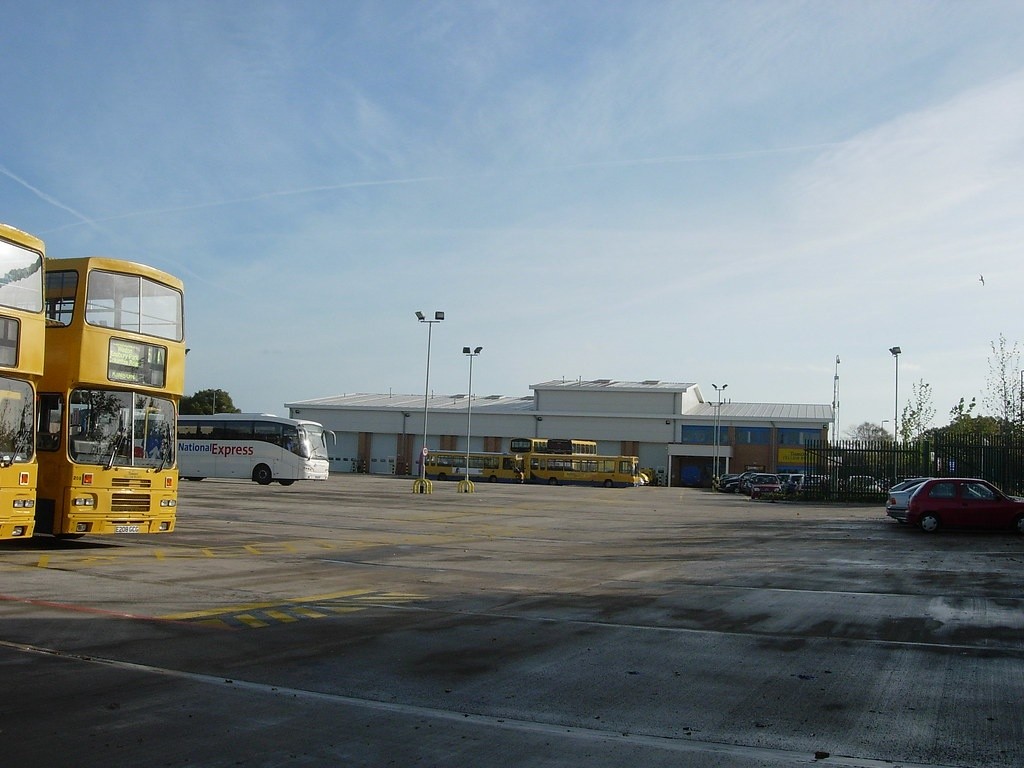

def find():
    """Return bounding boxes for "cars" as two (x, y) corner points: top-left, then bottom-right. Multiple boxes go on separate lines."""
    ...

(718, 472), (883, 502)
(885, 477), (1024, 536)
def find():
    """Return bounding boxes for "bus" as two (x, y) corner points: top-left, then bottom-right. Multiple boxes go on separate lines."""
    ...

(0, 223), (337, 540)
(418, 438), (653, 488)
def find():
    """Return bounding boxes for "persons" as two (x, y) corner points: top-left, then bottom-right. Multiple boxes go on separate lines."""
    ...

(286, 437), (295, 450)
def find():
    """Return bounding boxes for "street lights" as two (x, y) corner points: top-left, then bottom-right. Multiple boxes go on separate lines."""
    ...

(882, 347), (901, 486)
(457, 347), (483, 493)
(832, 354), (840, 446)
(708, 384), (730, 493)
(401, 413), (410, 475)
(533, 415), (542, 438)
(413, 311), (444, 493)
